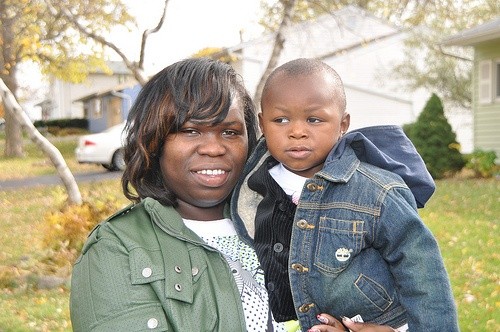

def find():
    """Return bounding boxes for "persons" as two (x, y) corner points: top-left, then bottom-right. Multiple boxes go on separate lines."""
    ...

(231, 59), (459, 332)
(70, 57), (397, 332)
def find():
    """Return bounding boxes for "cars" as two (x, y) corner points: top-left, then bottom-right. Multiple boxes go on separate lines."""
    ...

(75, 118), (135, 172)
